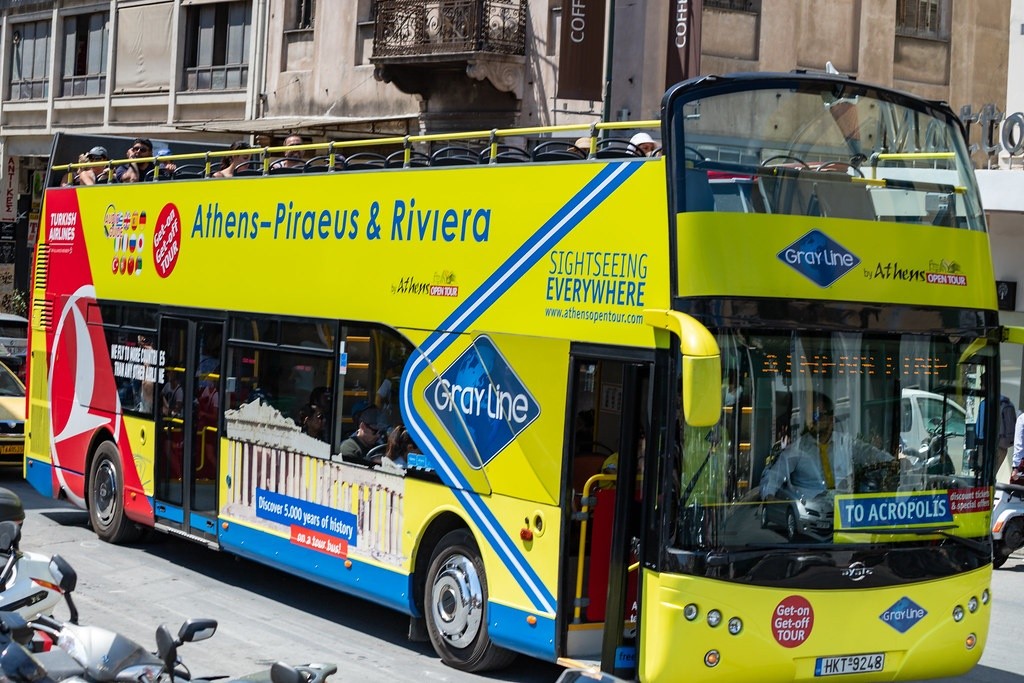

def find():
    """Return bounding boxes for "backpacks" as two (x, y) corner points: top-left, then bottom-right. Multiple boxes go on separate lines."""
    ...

(997, 397), (1017, 449)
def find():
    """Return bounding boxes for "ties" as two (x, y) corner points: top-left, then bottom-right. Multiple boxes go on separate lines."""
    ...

(814, 442), (835, 489)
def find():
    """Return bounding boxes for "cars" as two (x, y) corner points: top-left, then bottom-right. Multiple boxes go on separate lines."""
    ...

(0, 360), (26, 470)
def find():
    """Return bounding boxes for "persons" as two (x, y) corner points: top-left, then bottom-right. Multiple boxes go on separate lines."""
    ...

(117, 139), (176, 183)
(310, 386), (329, 416)
(385, 425), (420, 470)
(297, 404), (326, 441)
(1011, 412), (1024, 498)
(270, 136), (307, 169)
(760, 392), (898, 501)
(79, 146), (119, 185)
(159, 368), (183, 479)
(340, 408), (387, 466)
(377, 361), (395, 422)
(60, 153), (89, 187)
(197, 380), (218, 479)
(212, 141), (251, 178)
(627, 132), (660, 157)
(567, 138), (600, 158)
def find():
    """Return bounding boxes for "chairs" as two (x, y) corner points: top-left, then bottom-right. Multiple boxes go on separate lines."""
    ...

(73, 135), (878, 222)
(364, 443), (389, 463)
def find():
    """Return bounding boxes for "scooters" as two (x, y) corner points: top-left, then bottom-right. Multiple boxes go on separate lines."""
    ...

(990, 482), (1024, 569)
(0, 486), (337, 683)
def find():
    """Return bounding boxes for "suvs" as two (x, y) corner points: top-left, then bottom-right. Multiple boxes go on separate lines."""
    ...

(0, 312), (28, 358)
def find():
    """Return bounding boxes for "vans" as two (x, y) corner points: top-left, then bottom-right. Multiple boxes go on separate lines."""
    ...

(790, 384), (966, 476)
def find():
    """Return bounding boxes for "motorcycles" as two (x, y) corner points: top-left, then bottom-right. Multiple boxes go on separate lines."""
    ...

(899, 410), (956, 476)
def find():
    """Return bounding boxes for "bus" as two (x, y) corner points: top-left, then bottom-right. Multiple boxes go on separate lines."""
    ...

(23, 62), (1024, 683)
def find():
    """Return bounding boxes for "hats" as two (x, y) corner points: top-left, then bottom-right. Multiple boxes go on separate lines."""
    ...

(567, 137), (599, 153)
(626, 133), (660, 156)
(87, 147), (108, 160)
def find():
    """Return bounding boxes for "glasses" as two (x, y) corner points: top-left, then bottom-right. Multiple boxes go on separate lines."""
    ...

(132, 148), (148, 153)
(89, 156), (101, 162)
(798, 411), (834, 423)
(365, 424), (385, 436)
